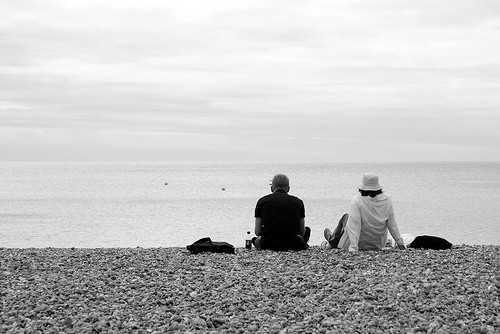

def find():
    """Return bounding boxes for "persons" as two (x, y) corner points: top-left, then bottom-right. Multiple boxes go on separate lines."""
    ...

(254, 174), (311, 250)
(323, 172), (405, 252)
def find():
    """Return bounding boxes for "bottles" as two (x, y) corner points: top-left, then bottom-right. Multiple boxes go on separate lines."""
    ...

(246, 231), (252, 250)
(385, 232), (392, 250)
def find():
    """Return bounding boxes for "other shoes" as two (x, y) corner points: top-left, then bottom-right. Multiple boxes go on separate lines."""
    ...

(324, 228), (335, 247)
(304, 227), (310, 241)
(252, 237), (258, 247)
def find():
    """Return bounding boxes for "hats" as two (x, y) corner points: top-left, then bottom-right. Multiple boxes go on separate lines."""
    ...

(358, 173), (382, 191)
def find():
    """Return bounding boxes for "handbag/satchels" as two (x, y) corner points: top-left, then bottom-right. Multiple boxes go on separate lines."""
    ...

(406, 236), (453, 250)
(187, 237), (235, 255)
